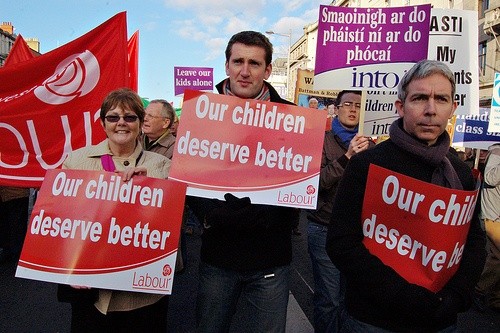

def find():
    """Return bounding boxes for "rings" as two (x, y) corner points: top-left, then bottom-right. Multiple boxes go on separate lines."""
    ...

(357, 145), (362, 149)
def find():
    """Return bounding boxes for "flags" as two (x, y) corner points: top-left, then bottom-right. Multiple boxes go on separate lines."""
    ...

(0, 10), (139, 186)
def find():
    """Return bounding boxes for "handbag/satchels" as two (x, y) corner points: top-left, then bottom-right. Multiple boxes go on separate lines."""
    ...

(58, 284), (99, 304)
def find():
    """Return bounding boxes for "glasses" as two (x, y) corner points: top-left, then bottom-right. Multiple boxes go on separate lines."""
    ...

(337, 102), (360, 110)
(104, 115), (138, 122)
(144, 111), (169, 121)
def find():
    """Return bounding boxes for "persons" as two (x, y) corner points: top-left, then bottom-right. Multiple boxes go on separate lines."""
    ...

(185, 31), (301, 333)
(306, 90), (375, 333)
(325, 60), (487, 333)
(56, 87), (201, 333)
(454, 145), (500, 333)
